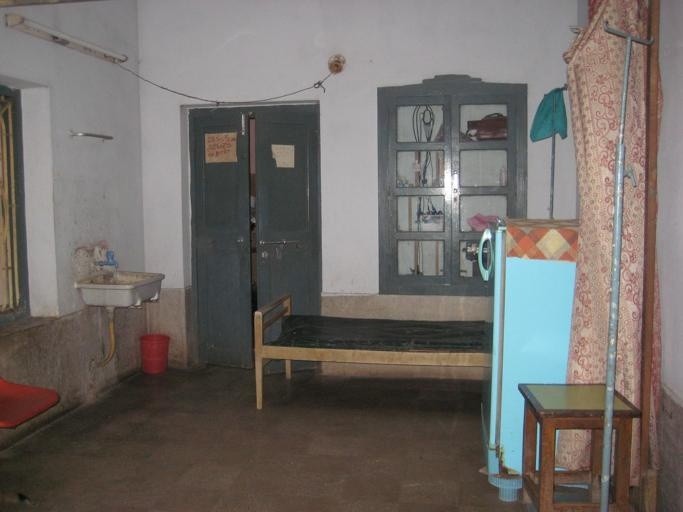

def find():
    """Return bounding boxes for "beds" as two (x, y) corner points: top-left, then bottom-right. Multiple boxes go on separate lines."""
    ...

(254, 292), (484, 409)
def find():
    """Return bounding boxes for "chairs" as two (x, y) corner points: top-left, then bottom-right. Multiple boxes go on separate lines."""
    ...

(0, 376), (60, 506)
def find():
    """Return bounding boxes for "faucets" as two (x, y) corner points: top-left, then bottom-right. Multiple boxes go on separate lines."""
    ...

(95, 250), (119, 270)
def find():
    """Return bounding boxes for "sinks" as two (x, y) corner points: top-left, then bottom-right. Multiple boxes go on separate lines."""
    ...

(73, 269), (165, 308)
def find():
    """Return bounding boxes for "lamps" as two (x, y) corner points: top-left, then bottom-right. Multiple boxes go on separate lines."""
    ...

(6, 13), (128, 64)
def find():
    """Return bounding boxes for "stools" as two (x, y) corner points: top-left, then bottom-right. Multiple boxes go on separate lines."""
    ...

(518, 384), (641, 512)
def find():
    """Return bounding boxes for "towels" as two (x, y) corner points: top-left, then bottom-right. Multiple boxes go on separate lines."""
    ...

(529, 88), (567, 141)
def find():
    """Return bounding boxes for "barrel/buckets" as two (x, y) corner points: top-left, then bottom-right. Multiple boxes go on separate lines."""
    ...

(140, 334), (169, 375)
